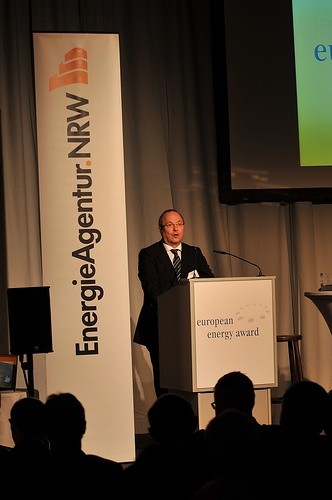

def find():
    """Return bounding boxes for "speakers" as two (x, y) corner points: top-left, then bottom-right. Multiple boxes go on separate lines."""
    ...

(6, 286), (54, 355)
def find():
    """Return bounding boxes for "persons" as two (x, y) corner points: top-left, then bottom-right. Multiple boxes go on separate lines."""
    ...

(0, 372), (332, 500)
(132, 209), (215, 398)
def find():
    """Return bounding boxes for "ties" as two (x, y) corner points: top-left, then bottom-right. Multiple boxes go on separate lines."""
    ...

(170, 249), (182, 284)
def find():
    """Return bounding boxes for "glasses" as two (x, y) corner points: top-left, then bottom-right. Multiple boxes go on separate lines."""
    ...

(210, 401), (217, 409)
(160, 222), (184, 229)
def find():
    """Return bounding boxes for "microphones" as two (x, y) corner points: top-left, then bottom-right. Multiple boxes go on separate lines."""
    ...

(213, 249), (265, 277)
